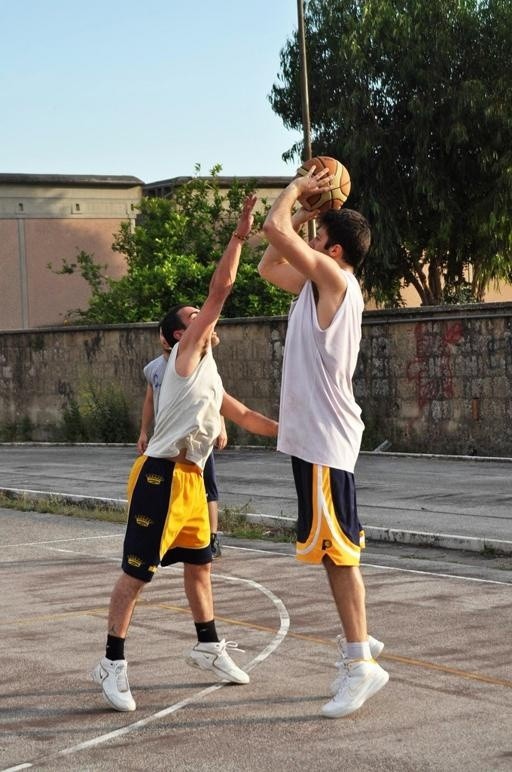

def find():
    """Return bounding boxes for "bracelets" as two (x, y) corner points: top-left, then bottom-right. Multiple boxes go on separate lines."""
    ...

(233, 230), (250, 241)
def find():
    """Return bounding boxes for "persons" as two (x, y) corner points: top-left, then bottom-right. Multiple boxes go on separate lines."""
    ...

(256, 163), (389, 719)
(137, 318), (228, 559)
(92, 191), (259, 712)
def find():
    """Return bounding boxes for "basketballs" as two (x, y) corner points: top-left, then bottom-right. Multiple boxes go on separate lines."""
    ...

(295, 156), (352, 216)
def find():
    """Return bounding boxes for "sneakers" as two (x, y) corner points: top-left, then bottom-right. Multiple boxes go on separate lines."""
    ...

(211, 538), (221, 559)
(93, 655), (137, 711)
(321, 658), (389, 718)
(329, 631), (385, 696)
(185, 638), (251, 684)
